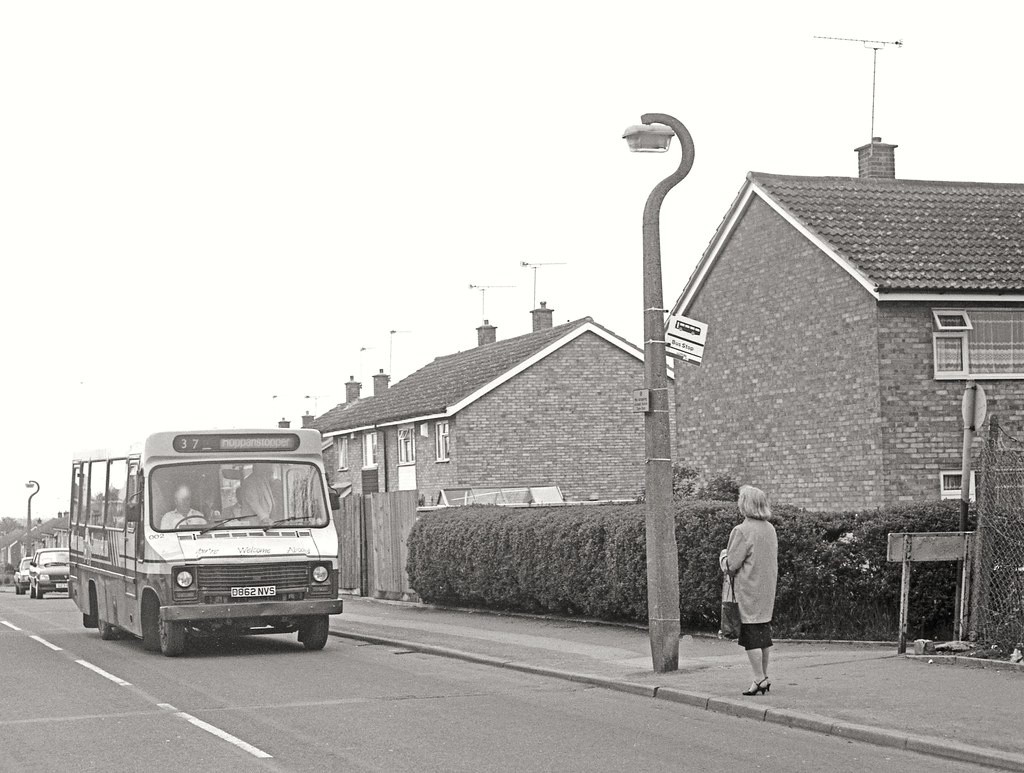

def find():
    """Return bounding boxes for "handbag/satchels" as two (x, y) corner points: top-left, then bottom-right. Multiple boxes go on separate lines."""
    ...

(722, 559), (741, 639)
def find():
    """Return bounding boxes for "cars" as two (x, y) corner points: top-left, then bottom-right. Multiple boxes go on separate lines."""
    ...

(13, 557), (33, 595)
(28, 548), (70, 600)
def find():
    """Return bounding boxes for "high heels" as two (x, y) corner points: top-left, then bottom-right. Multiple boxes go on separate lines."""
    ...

(742, 677), (767, 695)
(764, 678), (770, 692)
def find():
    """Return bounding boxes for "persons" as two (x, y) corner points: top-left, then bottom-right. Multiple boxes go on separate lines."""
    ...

(718, 483), (779, 697)
(220, 461), (284, 526)
(160, 484), (206, 529)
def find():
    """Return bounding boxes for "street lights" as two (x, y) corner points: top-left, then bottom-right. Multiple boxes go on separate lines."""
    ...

(25, 481), (39, 557)
(622, 113), (696, 675)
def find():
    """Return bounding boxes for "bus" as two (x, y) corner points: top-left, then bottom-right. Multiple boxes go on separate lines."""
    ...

(67, 428), (344, 656)
